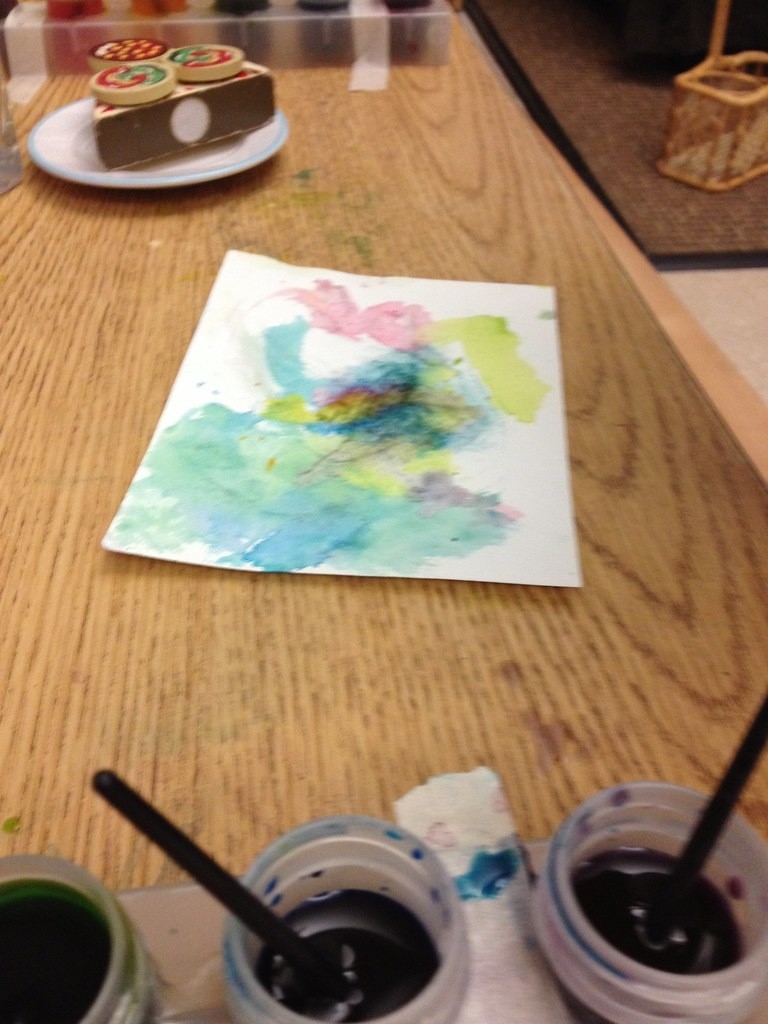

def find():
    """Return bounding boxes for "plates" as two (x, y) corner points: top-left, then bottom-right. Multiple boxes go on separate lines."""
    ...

(29, 99), (288, 189)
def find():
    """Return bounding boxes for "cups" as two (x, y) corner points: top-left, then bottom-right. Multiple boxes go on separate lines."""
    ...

(537, 784), (768, 1024)
(0, 855), (152, 1024)
(218, 814), (473, 1024)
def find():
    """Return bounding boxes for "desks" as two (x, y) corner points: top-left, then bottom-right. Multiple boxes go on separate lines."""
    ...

(0, 12), (768, 1024)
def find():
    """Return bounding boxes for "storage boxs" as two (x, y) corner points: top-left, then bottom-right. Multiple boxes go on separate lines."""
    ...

(3, 0), (455, 77)
